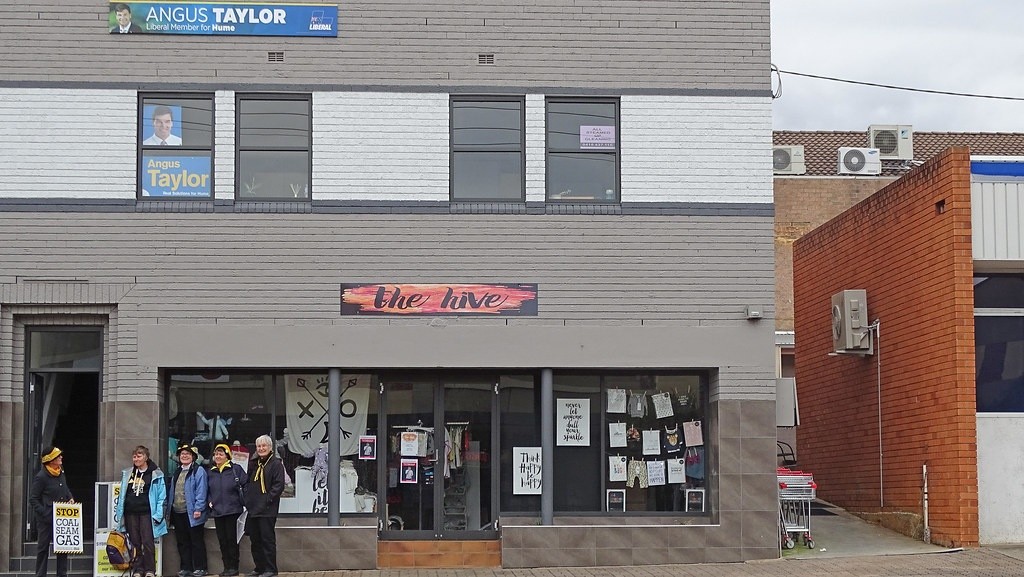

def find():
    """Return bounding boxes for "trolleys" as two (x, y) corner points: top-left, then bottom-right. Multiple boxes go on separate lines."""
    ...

(777, 466), (816, 549)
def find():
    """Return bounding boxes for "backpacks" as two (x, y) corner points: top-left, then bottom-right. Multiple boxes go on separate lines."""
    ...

(105, 527), (134, 571)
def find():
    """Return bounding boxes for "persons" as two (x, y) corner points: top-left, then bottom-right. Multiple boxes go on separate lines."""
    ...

(111, 3), (142, 32)
(405, 467), (413, 481)
(206, 443), (249, 577)
(117, 445), (168, 577)
(197, 412), (229, 440)
(166, 443), (210, 577)
(29, 446), (75, 577)
(143, 107), (182, 145)
(363, 442), (372, 456)
(244, 434), (285, 577)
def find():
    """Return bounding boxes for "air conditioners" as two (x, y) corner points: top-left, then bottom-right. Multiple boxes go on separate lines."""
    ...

(867, 124), (914, 162)
(772, 144), (807, 176)
(838, 147), (882, 176)
(830, 289), (869, 350)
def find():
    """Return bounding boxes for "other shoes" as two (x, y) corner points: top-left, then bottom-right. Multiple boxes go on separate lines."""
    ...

(176, 569), (193, 577)
(219, 569), (239, 577)
(245, 570), (264, 577)
(258, 571), (275, 577)
(132, 569), (144, 577)
(143, 570), (155, 577)
(192, 569), (209, 577)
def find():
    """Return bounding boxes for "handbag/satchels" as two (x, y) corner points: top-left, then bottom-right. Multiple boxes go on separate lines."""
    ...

(232, 464), (246, 506)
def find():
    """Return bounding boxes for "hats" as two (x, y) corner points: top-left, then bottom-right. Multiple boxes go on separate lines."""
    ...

(41, 446), (62, 464)
(214, 443), (232, 461)
(176, 443), (199, 462)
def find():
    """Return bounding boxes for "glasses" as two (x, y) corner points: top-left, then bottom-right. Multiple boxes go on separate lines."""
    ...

(180, 452), (192, 456)
